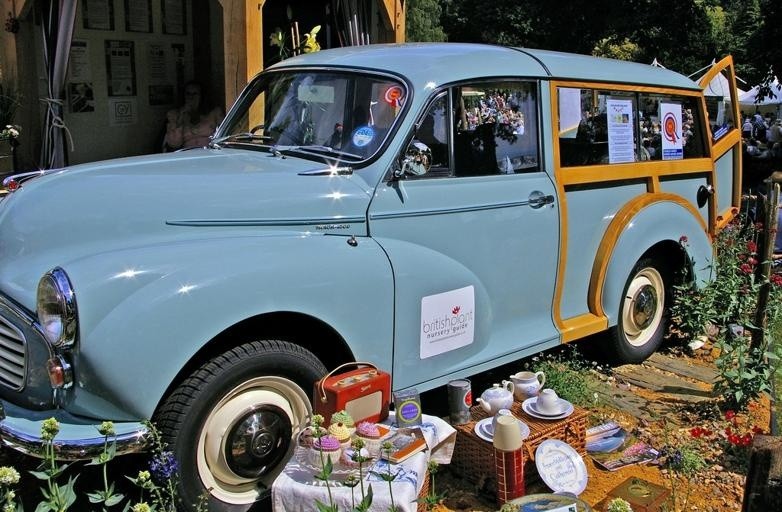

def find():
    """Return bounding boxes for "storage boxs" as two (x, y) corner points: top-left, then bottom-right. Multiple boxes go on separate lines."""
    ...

(269, 407), (457, 512)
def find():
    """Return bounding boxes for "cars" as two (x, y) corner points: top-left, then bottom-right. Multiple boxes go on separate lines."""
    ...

(0, 41), (743, 512)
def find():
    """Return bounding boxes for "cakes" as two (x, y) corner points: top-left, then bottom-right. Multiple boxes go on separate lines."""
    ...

(355, 422), (380, 440)
(299, 426), (326, 449)
(341, 447), (372, 470)
(327, 422), (351, 453)
(330, 410), (356, 435)
(308, 435), (341, 469)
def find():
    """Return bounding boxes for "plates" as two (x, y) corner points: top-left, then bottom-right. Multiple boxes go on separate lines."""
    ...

(521, 397), (574, 420)
(499, 493), (592, 512)
(481, 418), (527, 435)
(528, 398), (570, 415)
(534, 439), (588, 494)
(475, 416), (530, 441)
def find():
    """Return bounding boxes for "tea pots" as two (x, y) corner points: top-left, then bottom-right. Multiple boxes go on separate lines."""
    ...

(509, 369), (544, 401)
(476, 380), (514, 415)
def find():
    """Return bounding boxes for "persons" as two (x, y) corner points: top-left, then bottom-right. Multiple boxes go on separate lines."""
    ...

(462, 93), (525, 135)
(632, 108), (697, 159)
(742, 111), (782, 159)
(160, 80), (225, 153)
(750, 172), (782, 254)
(580, 106), (601, 136)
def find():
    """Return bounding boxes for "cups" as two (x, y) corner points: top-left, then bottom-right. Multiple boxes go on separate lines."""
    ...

(494, 417), (526, 506)
(537, 389), (559, 413)
(490, 408), (513, 434)
